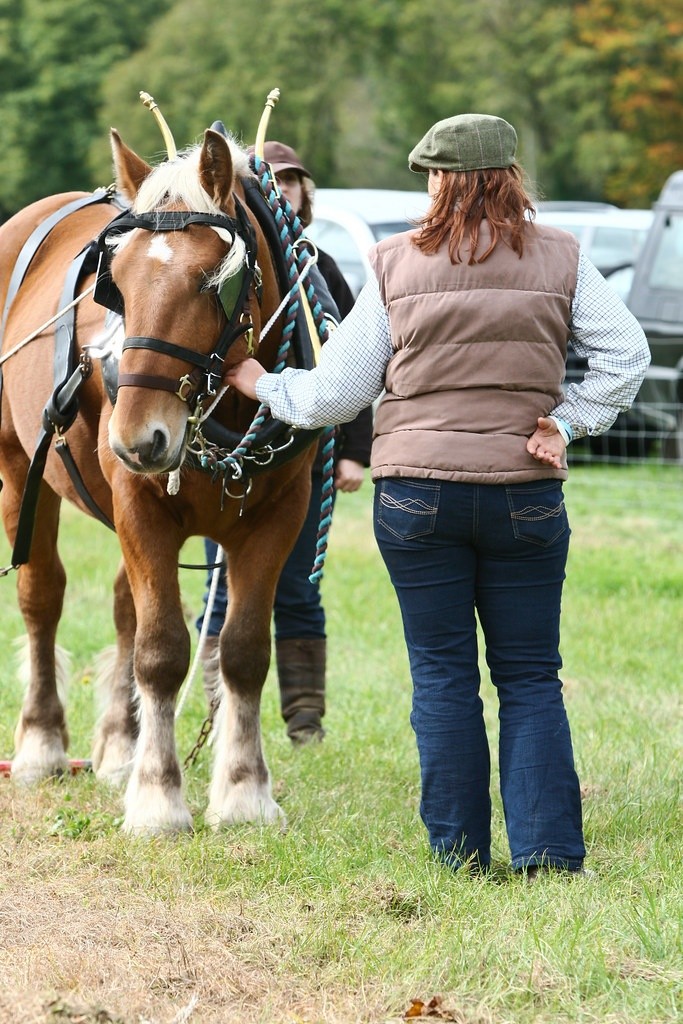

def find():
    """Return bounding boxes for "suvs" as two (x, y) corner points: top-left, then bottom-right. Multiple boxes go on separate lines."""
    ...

(626, 169), (683, 462)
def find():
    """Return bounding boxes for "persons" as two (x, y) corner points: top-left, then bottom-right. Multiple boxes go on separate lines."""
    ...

(196, 143), (374, 746)
(222, 115), (652, 880)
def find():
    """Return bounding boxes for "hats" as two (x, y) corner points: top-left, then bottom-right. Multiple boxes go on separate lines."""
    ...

(408, 114), (518, 174)
(249, 140), (312, 178)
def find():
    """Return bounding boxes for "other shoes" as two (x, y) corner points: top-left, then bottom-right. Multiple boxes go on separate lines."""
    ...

(527, 864), (599, 891)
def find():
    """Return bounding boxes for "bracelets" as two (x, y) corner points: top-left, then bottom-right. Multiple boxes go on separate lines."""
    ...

(548, 415), (569, 446)
(559, 421), (573, 442)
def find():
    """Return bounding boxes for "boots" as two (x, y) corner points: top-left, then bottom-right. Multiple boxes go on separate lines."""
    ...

(201, 635), (220, 717)
(275, 638), (326, 749)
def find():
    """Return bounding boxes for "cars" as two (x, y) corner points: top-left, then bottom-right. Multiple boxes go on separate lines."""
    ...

(301, 188), (653, 370)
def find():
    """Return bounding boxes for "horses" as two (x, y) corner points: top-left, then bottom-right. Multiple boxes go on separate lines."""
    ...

(0, 127), (319, 837)
